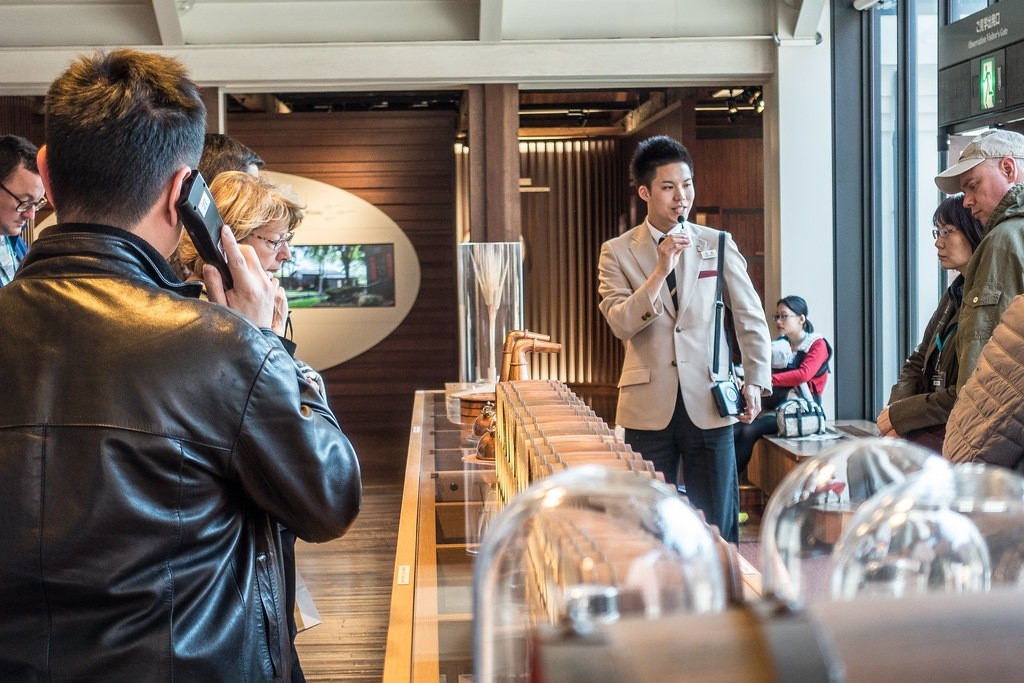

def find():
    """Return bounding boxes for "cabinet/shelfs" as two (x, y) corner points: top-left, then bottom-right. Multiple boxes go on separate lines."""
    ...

(382, 390), (526, 683)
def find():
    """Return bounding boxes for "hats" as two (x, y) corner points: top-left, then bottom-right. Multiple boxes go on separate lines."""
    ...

(934, 130), (1024, 194)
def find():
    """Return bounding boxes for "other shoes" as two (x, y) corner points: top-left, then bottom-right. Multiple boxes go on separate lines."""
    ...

(739, 512), (750, 526)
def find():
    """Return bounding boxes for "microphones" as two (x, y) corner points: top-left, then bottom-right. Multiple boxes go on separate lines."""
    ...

(677, 215), (685, 229)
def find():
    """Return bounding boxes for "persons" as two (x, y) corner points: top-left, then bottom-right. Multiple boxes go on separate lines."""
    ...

(597, 135), (773, 553)
(677, 295), (832, 498)
(0, 134), (45, 289)
(164, 132), (327, 406)
(876, 129), (1024, 474)
(0, 48), (362, 683)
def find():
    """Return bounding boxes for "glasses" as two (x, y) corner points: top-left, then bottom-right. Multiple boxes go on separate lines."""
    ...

(0, 183), (47, 212)
(771, 311), (799, 322)
(932, 228), (959, 239)
(249, 231), (295, 252)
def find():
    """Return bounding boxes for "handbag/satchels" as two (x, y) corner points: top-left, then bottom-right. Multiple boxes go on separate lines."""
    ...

(711, 382), (746, 419)
(775, 397), (826, 438)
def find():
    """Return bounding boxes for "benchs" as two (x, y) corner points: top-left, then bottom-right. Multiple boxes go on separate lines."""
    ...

(746, 419), (882, 497)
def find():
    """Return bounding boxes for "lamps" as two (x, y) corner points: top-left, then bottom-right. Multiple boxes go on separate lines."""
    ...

(711, 87), (765, 124)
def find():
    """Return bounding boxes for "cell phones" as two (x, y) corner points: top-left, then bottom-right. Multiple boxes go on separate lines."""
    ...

(174, 169), (235, 294)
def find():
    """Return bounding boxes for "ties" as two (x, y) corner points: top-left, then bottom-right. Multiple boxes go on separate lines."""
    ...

(658, 237), (679, 313)
